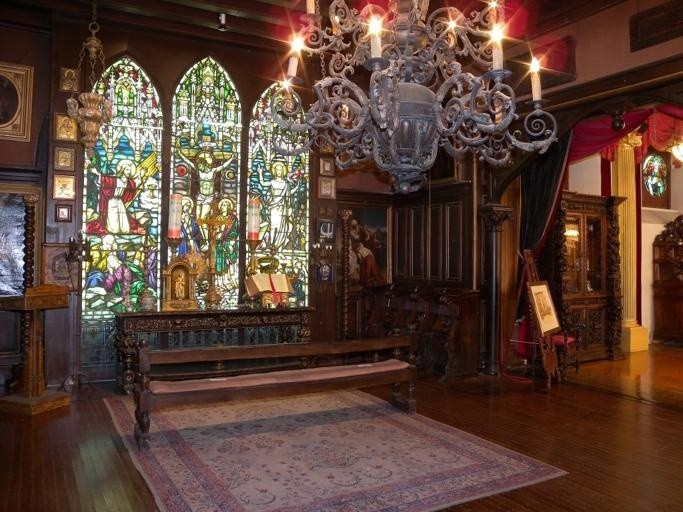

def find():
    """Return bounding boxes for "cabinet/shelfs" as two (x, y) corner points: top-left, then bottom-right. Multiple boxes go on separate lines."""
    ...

(540, 183), (627, 368)
(391, 184), (475, 288)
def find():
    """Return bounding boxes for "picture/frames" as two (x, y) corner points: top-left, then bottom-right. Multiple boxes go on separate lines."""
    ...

(526, 279), (563, 338)
(0, 59), (34, 144)
(318, 122), (337, 202)
(332, 186), (398, 295)
(50, 60), (80, 223)
(0, 182), (47, 299)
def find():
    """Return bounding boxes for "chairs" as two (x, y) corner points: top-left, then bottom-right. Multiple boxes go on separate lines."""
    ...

(527, 295), (580, 381)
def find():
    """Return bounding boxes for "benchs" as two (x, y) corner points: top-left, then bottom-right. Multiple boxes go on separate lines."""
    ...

(132, 321), (427, 453)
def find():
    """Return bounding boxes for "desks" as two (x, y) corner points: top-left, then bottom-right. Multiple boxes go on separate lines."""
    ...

(110, 304), (317, 398)
(0, 285), (75, 415)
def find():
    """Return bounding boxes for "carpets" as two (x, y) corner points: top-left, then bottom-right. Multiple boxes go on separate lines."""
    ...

(99, 373), (572, 509)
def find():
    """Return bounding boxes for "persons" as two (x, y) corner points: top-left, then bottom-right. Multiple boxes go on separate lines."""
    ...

(348, 218), (383, 287)
(85, 147), (307, 315)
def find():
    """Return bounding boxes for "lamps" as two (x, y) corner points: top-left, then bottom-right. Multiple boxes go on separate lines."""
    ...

(261, 1), (561, 200)
(246, 198), (259, 240)
(64, 1), (112, 163)
(168, 192), (182, 238)
